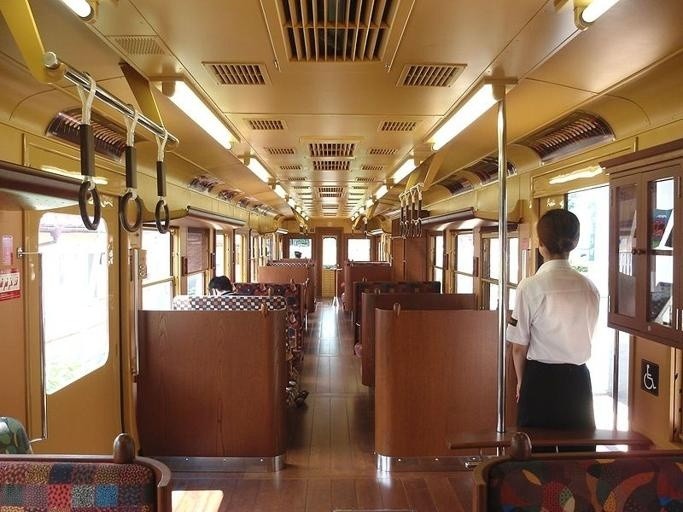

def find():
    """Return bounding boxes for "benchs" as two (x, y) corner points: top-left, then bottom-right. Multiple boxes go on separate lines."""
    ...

(0, 433), (171, 511)
(334, 261), (501, 472)
(139, 260), (317, 473)
(470, 449), (683, 511)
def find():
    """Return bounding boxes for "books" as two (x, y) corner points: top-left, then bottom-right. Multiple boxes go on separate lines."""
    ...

(628, 209), (672, 249)
(650, 282), (672, 324)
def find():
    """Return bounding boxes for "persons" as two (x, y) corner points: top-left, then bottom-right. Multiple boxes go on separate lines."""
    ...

(294, 251), (302, 259)
(504, 208), (601, 452)
(208, 276), (234, 294)
